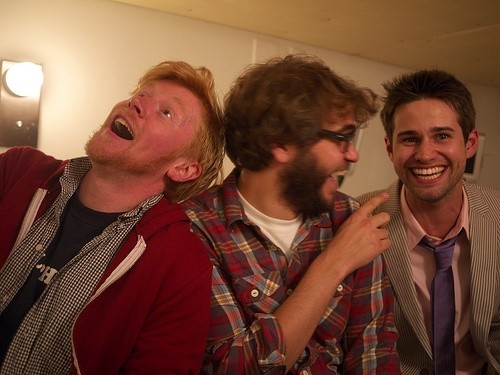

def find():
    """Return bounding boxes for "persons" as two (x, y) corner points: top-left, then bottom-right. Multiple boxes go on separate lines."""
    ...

(0, 60), (226, 375)
(353, 69), (500, 375)
(178, 55), (401, 375)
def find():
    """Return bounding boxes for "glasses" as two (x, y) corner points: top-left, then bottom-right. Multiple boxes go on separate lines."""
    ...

(317, 128), (355, 154)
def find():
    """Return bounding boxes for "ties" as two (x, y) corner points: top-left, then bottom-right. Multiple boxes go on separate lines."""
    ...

(417, 232), (460, 375)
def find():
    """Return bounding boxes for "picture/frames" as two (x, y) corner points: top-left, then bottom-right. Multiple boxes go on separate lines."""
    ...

(463, 133), (486, 184)
